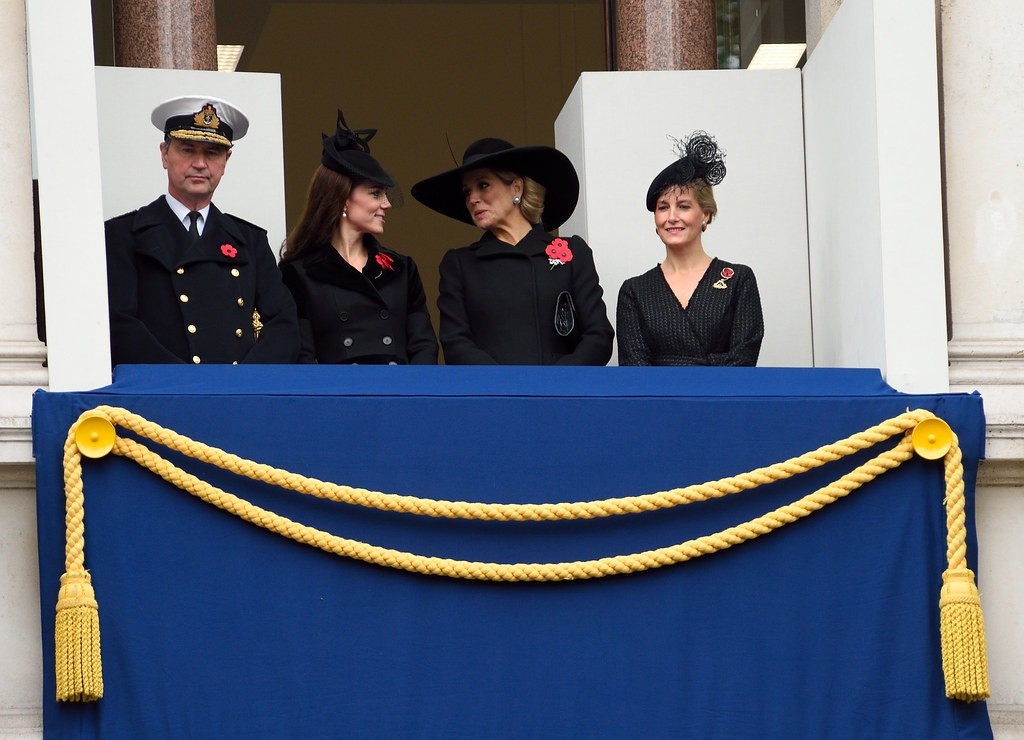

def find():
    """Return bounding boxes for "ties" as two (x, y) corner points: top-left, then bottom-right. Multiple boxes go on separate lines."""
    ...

(187, 211), (202, 238)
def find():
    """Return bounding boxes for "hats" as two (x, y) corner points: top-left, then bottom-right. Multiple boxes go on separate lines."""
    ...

(646, 130), (728, 212)
(411, 138), (579, 231)
(322, 109), (405, 207)
(151, 96), (250, 147)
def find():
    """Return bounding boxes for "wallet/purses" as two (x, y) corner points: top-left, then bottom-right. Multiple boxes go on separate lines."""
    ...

(554, 291), (577, 336)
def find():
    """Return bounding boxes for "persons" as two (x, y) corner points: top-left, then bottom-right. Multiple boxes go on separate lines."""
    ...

(279, 136), (439, 364)
(410, 137), (614, 366)
(104, 96), (297, 363)
(616, 156), (764, 366)
